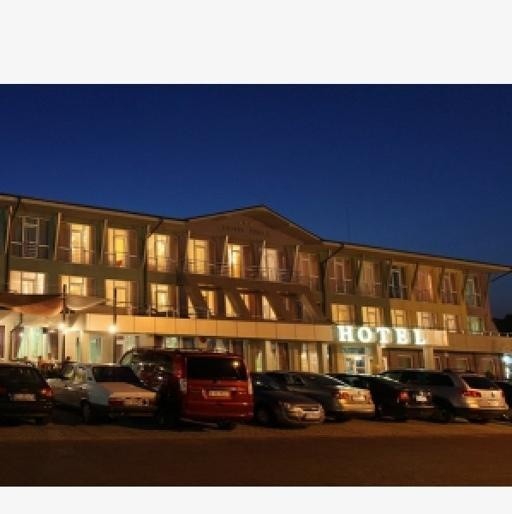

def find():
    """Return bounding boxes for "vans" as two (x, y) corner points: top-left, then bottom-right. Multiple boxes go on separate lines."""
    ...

(112, 346), (257, 434)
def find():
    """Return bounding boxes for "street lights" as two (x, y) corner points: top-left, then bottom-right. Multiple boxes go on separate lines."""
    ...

(110, 324), (120, 365)
(47, 320), (82, 367)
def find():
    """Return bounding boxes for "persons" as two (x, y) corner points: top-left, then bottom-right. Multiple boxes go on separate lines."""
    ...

(45, 352), (54, 371)
(36, 355), (52, 376)
(56, 355), (73, 379)
